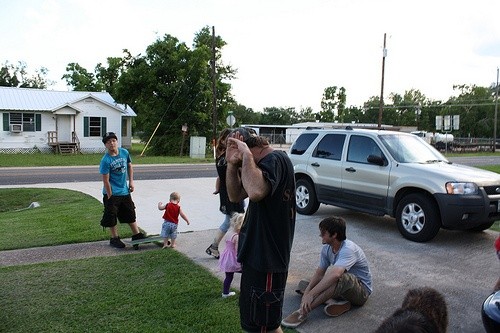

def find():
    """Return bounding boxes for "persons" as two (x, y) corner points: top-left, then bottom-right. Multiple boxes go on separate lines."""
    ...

(158, 193), (192, 250)
(217, 196), (248, 297)
(375, 309), (440, 333)
(481, 291), (500, 333)
(205, 128), (235, 259)
(99, 132), (152, 249)
(493, 235), (500, 290)
(281, 216), (373, 328)
(402, 286), (449, 333)
(225, 131), (296, 332)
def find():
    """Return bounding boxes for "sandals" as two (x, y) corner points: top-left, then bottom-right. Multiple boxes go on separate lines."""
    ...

(206, 243), (220, 258)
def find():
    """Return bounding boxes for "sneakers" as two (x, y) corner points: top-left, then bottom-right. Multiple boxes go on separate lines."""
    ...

(110, 236), (125, 248)
(132, 232), (151, 243)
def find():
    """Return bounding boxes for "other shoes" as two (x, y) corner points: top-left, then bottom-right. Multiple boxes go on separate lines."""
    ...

(221, 291), (236, 298)
(324, 300), (352, 316)
(281, 308), (310, 327)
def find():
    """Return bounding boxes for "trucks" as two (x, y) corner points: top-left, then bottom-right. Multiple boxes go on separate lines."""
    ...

(411, 131), (454, 153)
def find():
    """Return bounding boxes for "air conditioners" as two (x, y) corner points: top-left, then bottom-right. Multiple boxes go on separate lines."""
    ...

(10, 124), (23, 133)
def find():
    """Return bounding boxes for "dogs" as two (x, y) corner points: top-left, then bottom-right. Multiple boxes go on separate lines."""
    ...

(372, 287), (448, 333)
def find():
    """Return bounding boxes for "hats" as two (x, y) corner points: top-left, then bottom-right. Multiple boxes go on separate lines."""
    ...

(102, 131), (117, 143)
(216, 126), (258, 158)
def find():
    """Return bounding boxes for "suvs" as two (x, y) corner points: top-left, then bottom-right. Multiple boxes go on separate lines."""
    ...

(287, 126), (499, 243)
